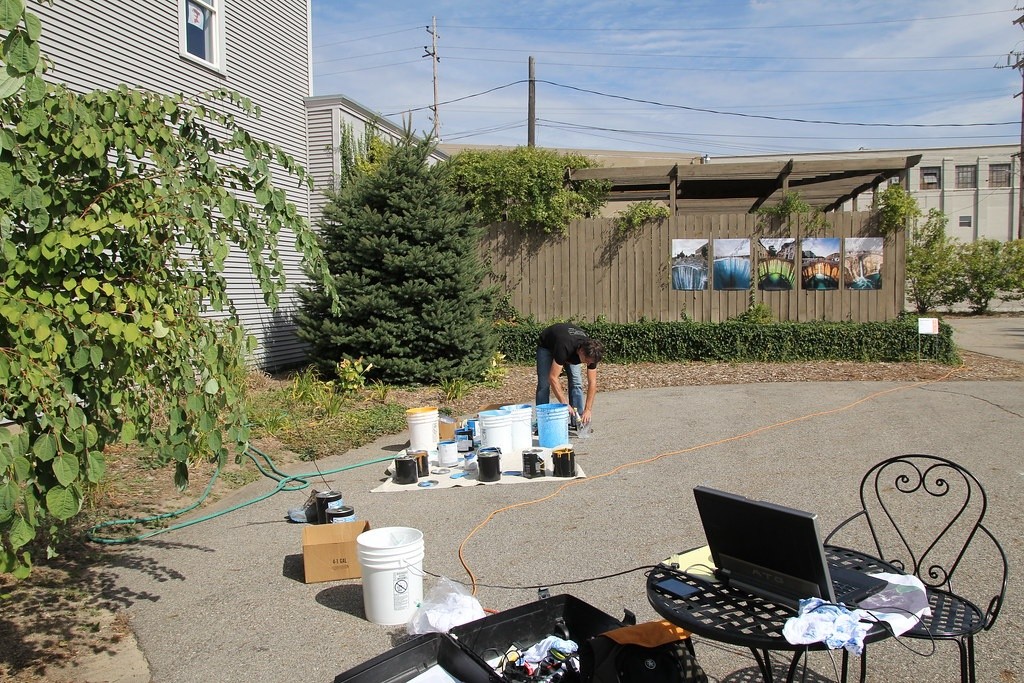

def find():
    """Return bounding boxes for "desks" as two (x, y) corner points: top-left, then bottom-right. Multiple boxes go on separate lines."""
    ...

(645, 543), (925, 683)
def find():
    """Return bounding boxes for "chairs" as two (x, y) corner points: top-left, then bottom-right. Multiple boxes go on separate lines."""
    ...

(822, 454), (1008, 683)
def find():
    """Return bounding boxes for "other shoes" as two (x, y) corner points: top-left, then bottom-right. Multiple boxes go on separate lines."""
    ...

(288, 489), (318, 521)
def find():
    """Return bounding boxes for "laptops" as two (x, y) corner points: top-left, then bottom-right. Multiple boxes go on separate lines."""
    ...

(693, 485), (888, 617)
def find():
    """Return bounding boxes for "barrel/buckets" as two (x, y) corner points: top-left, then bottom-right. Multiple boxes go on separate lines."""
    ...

(395, 455), (418, 485)
(325, 505), (355, 524)
(536, 403), (569, 448)
(522, 448), (546, 479)
(477, 403), (533, 482)
(406, 407), (440, 452)
(551, 448), (576, 478)
(467, 418), (480, 438)
(454, 429), (474, 452)
(315, 491), (343, 524)
(357, 527), (426, 626)
(438, 441), (459, 467)
(407, 450), (429, 477)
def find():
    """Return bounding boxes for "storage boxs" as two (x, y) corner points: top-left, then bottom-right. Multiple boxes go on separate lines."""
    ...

(334, 593), (636, 683)
(302, 519), (372, 584)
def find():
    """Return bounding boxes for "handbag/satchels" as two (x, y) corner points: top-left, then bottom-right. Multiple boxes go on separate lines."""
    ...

(579, 621), (709, 683)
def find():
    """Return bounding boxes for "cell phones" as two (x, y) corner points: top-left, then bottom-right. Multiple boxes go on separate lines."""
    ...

(653, 579), (702, 601)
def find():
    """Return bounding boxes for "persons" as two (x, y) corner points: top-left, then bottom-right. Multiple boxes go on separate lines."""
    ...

(534, 323), (604, 436)
